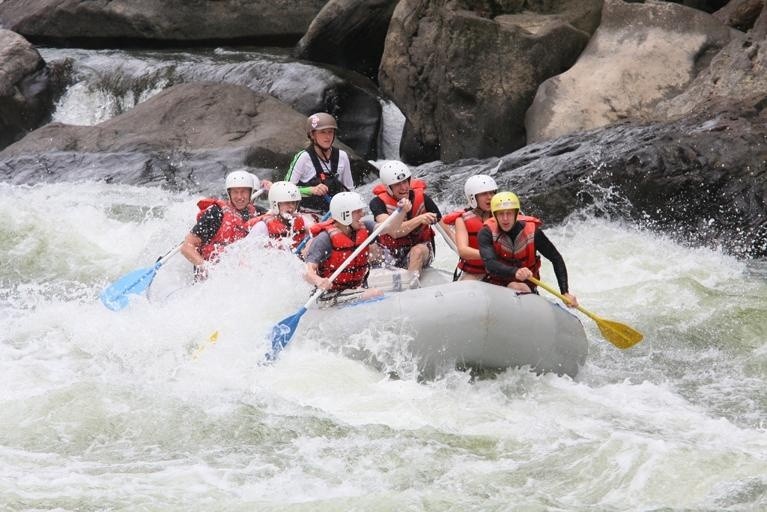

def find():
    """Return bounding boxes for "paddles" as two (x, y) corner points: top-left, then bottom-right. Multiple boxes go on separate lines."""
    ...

(272, 200), (404, 353)
(100, 188), (264, 311)
(527, 278), (643, 348)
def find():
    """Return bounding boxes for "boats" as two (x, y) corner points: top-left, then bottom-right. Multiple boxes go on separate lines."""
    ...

(146, 241), (588, 383)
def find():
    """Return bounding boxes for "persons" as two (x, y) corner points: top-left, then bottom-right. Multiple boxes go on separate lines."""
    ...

(259, 181), (313, 261)
(299, 190), (412, 309)
(476, 190), (579, 309)
(359, 158), (456, 290)
(452, 174), (499, 282)
(282, 112), (355, 219)
(249, 173), (267, 215)
(179, 170), (258, 282)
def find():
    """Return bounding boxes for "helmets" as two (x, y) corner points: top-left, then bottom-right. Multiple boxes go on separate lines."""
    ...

(268, 181), (303, 213)
(225, 170), (261, 208)
(330, 191), (368, 226)
(464, 174), (498, 210)
(380, 160), (412, 197)
(491, 191), (520, 228)
(307, 113), (338, 134)
